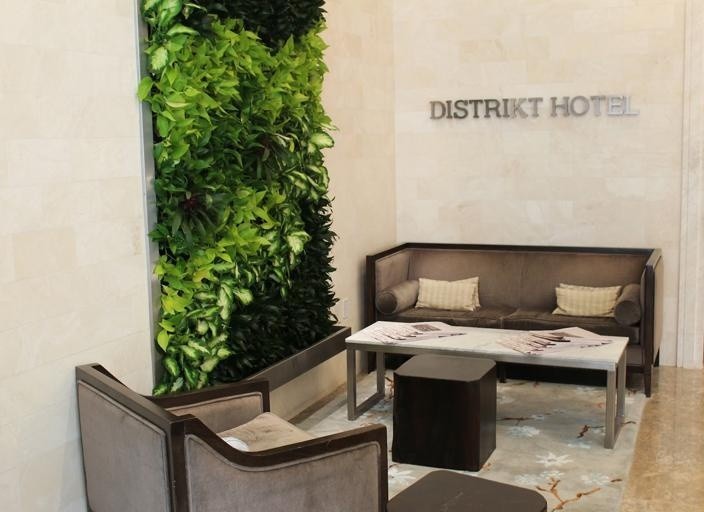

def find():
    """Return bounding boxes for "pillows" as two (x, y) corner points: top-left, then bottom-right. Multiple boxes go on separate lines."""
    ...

(416, 278), (475, 312)
(558, 282), (622, 298)
(553, 288), (617, 318)
(375, 279), (418, 314)
(614, 284), (642, 326)
(450, 275), (481, 308)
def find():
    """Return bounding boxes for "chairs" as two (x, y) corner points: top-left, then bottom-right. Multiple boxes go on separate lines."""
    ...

(76, 362), (388, 512)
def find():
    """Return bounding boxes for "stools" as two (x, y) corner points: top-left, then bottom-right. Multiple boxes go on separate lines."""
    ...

(387, 470), (547, 511)
(390, 353), (497, 472)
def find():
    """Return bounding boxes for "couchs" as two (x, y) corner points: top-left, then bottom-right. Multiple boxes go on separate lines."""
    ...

(366, 241), (664, 398)
